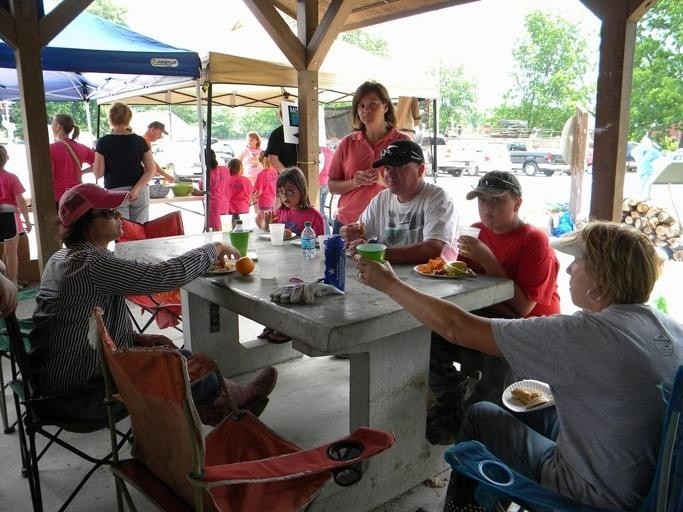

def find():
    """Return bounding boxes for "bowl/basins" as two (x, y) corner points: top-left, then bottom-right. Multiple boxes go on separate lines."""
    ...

(149, 185), (171, 198)
(171, 184), (193, 196)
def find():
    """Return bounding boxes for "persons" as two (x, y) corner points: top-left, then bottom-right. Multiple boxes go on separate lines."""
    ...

(0, 259), (18, 320)
(340, 141), (462, 265)
(198, 100), (335, 344)
(0, 102), (174, 292)
(327, 81), (413, 235)
(426, 170), (561, 445)
(355, 218), (683, 512)
(0, 183), (277, 433)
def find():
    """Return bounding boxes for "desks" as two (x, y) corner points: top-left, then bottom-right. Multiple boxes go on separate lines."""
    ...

(150, 196), (205, 219)
(147, 196), (206, 233)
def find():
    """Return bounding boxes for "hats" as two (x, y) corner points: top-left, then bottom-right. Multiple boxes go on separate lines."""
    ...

(373, 141), (424, 168)
(467, 171), (521, 199)
(551, 232), (586, 258)
(58, 183), (129, 229)
(149, 121), (168, 135)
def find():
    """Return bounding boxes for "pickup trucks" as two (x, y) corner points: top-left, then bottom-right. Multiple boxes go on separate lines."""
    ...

(508, 140), (573, 176)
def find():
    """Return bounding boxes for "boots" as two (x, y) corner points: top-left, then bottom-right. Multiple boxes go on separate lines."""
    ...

(201, 367), (277, 427)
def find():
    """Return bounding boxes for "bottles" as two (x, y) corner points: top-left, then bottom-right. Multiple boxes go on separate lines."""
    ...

(323, 234), (346, 291)
(230, 220), (248, 233)
(301, 222), (316, 260)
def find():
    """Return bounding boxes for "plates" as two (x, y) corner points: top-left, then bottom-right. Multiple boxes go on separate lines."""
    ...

(289, 239), (320, 246)
(204, 258), (240, 274)
(223, 249), (258, 259)
(502, 379), (555, 413)
(413, 263), (476, 278)
(257, 232), (296, 239)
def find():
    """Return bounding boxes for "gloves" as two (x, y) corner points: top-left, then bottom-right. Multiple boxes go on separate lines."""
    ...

(271, 278), (344, 305)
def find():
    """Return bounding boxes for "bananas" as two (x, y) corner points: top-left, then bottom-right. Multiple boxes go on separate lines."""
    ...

(443, 261), (473, 276)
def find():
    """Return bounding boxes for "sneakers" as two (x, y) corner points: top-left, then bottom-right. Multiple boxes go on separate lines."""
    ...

(268, 331), (291, 344)
(257, 328), (272, 337)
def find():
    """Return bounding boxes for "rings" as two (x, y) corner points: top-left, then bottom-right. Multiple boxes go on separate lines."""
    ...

(358, 273), (362, 279)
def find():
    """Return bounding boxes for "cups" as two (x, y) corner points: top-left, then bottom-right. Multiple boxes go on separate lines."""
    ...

(356, 243), (388, 264)
(268, 223), (285, 246)
(230, 232), (249, 257)
(317, 235), (330, 262)
(258, 250), (278, 279)
(458, 225), (481, 253)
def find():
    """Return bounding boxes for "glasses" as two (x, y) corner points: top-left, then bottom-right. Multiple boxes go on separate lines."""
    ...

(93, 208), (117, 218)
(278, 189), (298, 197)
(480, 177), (521, 196)
(381, 145), (423, 160)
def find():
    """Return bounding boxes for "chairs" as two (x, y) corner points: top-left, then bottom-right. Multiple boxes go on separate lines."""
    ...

(0, 288), (48, 434)
(442, 364), (683, 512)
(91, 308), (396, 511)
(4, 306), (138, 511)
(114, 210), (186, 335)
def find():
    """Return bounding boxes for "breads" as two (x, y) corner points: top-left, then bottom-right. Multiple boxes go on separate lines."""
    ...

(511, 387), (548, 409)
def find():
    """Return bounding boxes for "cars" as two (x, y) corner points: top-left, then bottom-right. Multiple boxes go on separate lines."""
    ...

(148, 136), (236, 181)
(423, 134), (507, 175)
(623, 144), (644, 171)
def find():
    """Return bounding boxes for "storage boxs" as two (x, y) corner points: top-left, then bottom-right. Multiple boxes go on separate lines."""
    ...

(17, 259), (40, 281)
(0, 232), (30, 261)
